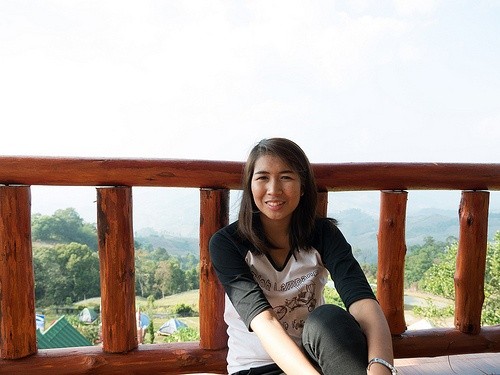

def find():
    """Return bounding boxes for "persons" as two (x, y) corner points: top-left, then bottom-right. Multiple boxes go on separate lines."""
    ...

(209, 138), (397, 375)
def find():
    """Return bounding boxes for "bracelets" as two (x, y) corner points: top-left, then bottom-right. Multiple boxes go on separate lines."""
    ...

(367, 358), (398, 375)
